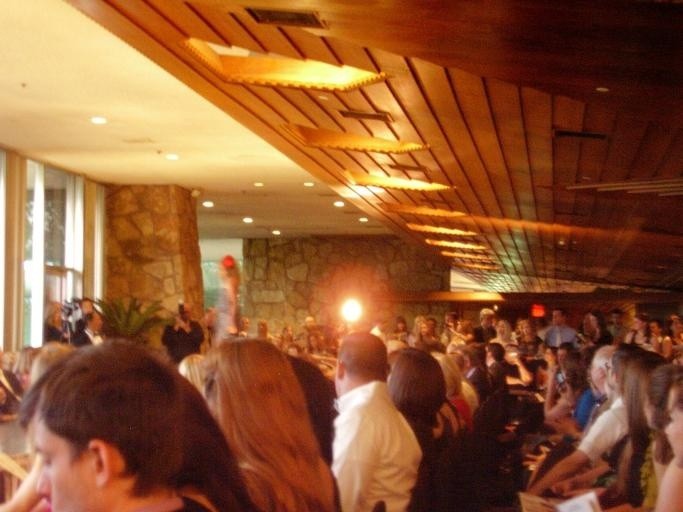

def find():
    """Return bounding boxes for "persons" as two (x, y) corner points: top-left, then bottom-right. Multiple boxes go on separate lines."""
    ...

(0, 254), (682, 512)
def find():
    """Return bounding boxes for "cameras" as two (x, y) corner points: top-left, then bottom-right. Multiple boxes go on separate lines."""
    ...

(177, 298), (188, 322)
(556, 370), (564, 385)
